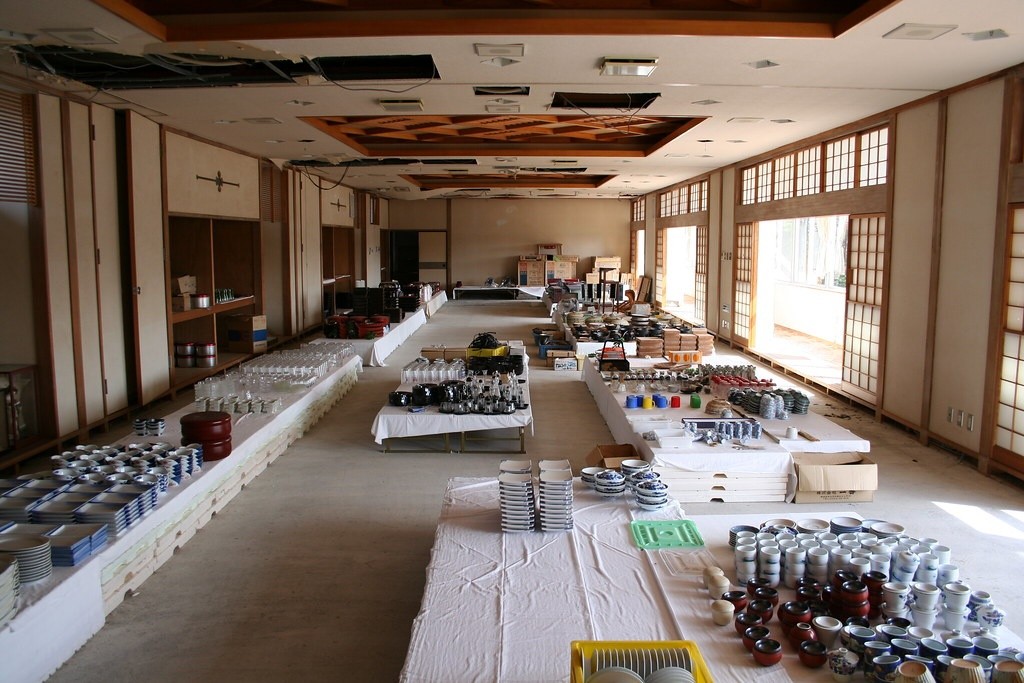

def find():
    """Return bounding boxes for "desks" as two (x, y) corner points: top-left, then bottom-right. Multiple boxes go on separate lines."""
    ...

(453, 286), (519, 300)
(370, 365), (535, 455)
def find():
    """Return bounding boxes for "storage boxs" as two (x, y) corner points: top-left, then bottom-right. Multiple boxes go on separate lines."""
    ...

(171, 275), (195, 310)
(517, 243), (651, 303)
(570, 638), (713, 683)
(586, 443), (642, 472)
(790, 450), (879, 504)
(636, 327), (715, 364)
(420, 340), (527, 362)
(529, 326), (584, 371)
(224, 314), (267, 354)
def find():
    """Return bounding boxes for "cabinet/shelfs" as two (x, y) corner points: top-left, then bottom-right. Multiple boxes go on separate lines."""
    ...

(166, 211), (263, 399)
(319, 223), (355, 323)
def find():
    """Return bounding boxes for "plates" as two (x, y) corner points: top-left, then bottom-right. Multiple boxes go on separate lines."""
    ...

(497, 458), (575, 534)
(193, 394), (282, 416)
(576, 646), (695, 683)
(0, 478), (160, 537)
(388, 380), (466, 407)
(0, 533), (53, 629)
(0, 521), (109, 567)
(727, 515), (907, 548)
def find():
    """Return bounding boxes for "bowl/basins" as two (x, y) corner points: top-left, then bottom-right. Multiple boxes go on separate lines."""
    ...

(580, 458), (669, 513)
(732, 530), (960, 584)
(727, 386), (810, 420)
(704, 398), (732, 414)
(813, 616), (935, 649)
(131, 417), (166, 437)
(49, 439), (204, 506)
(414, 356), (465, 367)
(862, 635), (1024, 683)
(721, 570), (888, 670)
(574, 322), (691, 343)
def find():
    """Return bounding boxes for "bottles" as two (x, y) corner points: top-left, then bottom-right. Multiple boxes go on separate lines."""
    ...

(710, 374), (777, 399)
(610, 369), (675, 393)
(677, 363), (757, 394)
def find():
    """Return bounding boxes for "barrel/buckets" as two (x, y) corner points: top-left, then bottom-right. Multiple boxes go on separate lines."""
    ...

(539, 333), (553, 345)
(879, 584), (972, 632)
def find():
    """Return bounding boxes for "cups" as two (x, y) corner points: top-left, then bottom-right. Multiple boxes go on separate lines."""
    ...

(400, 361), (461, 385)
(193, 341), (356, 401)
(720, 409), (734, 419)
(685, 420), (762, 444)
(785, 426), (798, 440)
(625, 392), (701, 409)
